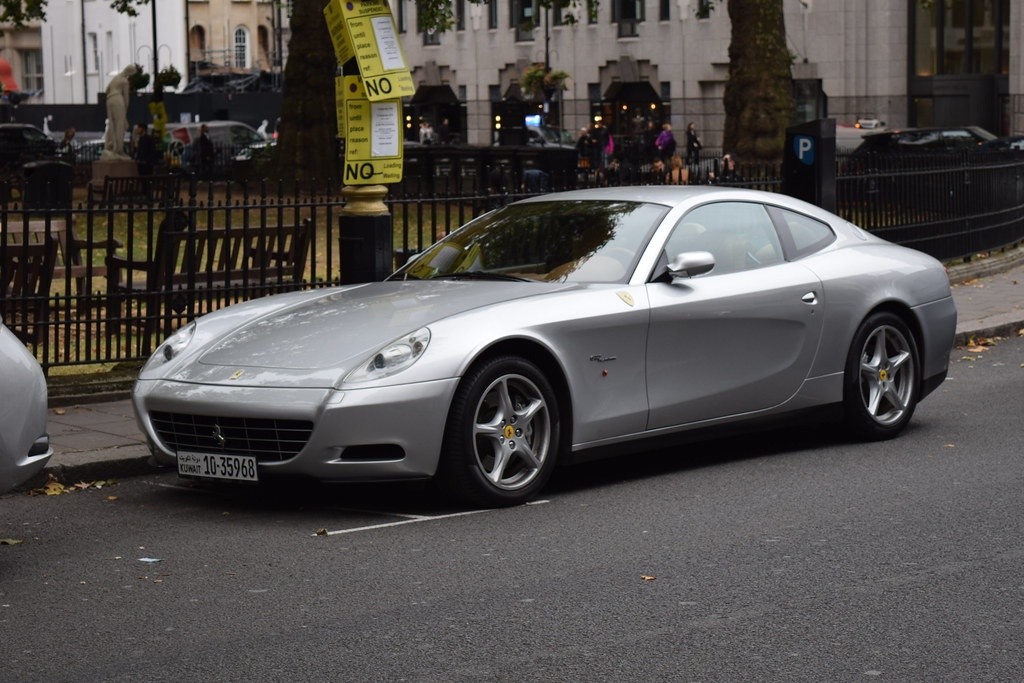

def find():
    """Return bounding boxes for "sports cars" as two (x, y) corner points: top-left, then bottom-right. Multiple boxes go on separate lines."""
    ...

(133, 184), (959, 509)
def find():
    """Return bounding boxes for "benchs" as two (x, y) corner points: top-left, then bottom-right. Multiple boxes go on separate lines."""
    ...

(104, 215), (316, 337)
(0, 235), (62, 344)
(91, 171), (175, 211)
(22, 173), (74, 210)
(0, 213), (124, 305)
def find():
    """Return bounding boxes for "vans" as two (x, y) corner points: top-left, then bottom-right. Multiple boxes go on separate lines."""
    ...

(146, 120), (266, 175)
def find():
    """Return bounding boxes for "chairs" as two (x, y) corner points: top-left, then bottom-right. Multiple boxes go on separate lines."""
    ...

(668, 221), (705, 255)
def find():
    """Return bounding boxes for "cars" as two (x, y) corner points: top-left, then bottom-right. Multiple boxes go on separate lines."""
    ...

(495, 126), (578, 188)
(838, 123), (1024, 205)
(0, 320), (55, 495)
(0, 122), (134, 188)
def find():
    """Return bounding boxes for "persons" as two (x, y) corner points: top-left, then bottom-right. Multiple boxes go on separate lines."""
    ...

(105, 64), (138, 155)
(438, 119), (452, 144)
(686, 123), (702, 165)
(655, 123), (676, 167)
(0, 93), (286, 182)
(576, 114), (663, 170)
(419, 121), (434, 144)
